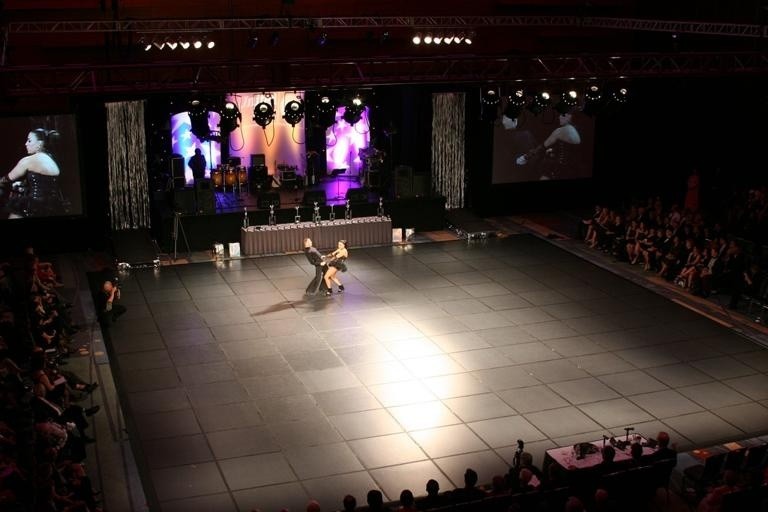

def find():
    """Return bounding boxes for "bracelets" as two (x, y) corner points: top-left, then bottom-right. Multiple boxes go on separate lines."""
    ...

(0, 173), (12, 183)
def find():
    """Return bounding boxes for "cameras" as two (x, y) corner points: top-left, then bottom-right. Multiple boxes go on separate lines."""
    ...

(114, 277), (122, 292)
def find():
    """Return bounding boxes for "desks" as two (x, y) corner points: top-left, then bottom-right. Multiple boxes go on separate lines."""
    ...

(545, 433), (661, 475)
(240, 216), (393, 255)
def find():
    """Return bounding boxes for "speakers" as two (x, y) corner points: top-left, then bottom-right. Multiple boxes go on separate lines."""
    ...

(304, 190), (326, 205)
(173, 187), (195, 216)
(345, 188), (368, 205)
(394, 165), (412, 201)
(257, 192), (280, 209)
(195, 178), (216, 216)
(412, 171), (431, 200)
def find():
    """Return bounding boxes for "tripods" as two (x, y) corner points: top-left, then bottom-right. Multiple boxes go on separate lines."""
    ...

(167, 215), (192, 260)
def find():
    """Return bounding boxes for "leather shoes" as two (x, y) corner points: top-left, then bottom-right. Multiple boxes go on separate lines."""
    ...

(83, 404), (100, 417)
(305, 285), (345, 297)
(80, 381), (99, 396)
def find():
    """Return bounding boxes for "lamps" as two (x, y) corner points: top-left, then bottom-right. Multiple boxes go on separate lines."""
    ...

(187, 93), (367, 143)
(480, 77), (630, 124)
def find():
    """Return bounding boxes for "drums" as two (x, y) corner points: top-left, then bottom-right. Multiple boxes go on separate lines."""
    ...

(225, 168), (237, 184)
(213, 171), (222, 187)
(217, 164), (225, 171)
(223, 164), (231, 171)
(237, 166), (247, 185)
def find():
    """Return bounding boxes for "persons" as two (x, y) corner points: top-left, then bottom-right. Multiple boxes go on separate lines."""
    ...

(308, 499), (320, 511)
(502, 112), (581, 180)
(565, 496), (583, 510)
(699, 467), (767, 510)
(624, 443), (652, 469)
(488, 452), (544, 496)
(592, 488), (613, 510)
(189, 148), (206, 184)
(584, 200), (768, 323)
(0, 128), (63, 219)
(650, 433), (678, 470)
(0, 256), (99, 512)
(452, 466), (488, 500)
(416, 479), (452, 510)
(304, 238), (348, 296)
(367, 490), (383, 511)
(342, 495), (356, 510)
(399, 490), (415, 511)
(94, 280), (127, 323)
(600, 446), (617, 476)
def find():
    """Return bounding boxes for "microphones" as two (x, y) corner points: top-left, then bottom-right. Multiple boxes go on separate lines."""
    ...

(625, 428), (634, 430)
(603, 435), (609, 440)
